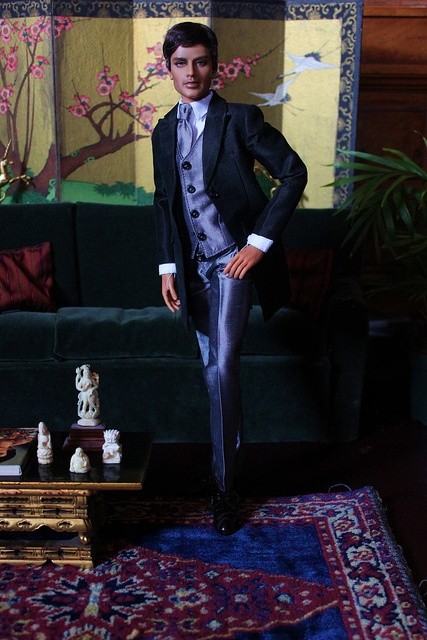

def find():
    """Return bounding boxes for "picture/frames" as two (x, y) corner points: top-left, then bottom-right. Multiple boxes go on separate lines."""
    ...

(0, 0), (365, 205)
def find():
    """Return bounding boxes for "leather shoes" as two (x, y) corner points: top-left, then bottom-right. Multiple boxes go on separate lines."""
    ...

(212, 487), (240, 536)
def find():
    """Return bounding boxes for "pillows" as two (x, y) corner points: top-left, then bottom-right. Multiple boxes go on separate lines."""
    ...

(0, 242), (55, 312)
(283, 245), (331, 306)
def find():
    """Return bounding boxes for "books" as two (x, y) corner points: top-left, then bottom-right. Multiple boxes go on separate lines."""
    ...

(0, 428), (38, 476)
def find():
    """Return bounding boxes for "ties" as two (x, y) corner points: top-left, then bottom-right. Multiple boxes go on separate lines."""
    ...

(176, 102), (193, 159)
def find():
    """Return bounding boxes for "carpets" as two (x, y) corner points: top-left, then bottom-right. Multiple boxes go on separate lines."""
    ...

(0, 484), (427, 638)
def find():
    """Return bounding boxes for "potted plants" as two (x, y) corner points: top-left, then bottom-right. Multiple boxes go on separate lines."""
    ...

(332, 131), (427, 426)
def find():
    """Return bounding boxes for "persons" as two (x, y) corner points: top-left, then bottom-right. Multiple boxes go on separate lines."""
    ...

(0, 428), (37, 462)
(38, 422), (52, 455)
(78, 367), (99, 413)
(70, 447), (89, 469)
(151, 23), (307, 534)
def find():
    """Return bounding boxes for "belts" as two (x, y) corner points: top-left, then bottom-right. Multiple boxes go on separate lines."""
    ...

(196, 247), (231, 262)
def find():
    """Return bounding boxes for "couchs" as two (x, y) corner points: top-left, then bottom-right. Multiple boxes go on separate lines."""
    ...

(0, 209), (387, 447)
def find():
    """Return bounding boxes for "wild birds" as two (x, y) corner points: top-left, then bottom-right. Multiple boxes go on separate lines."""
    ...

(247, 39), (342, 118)
